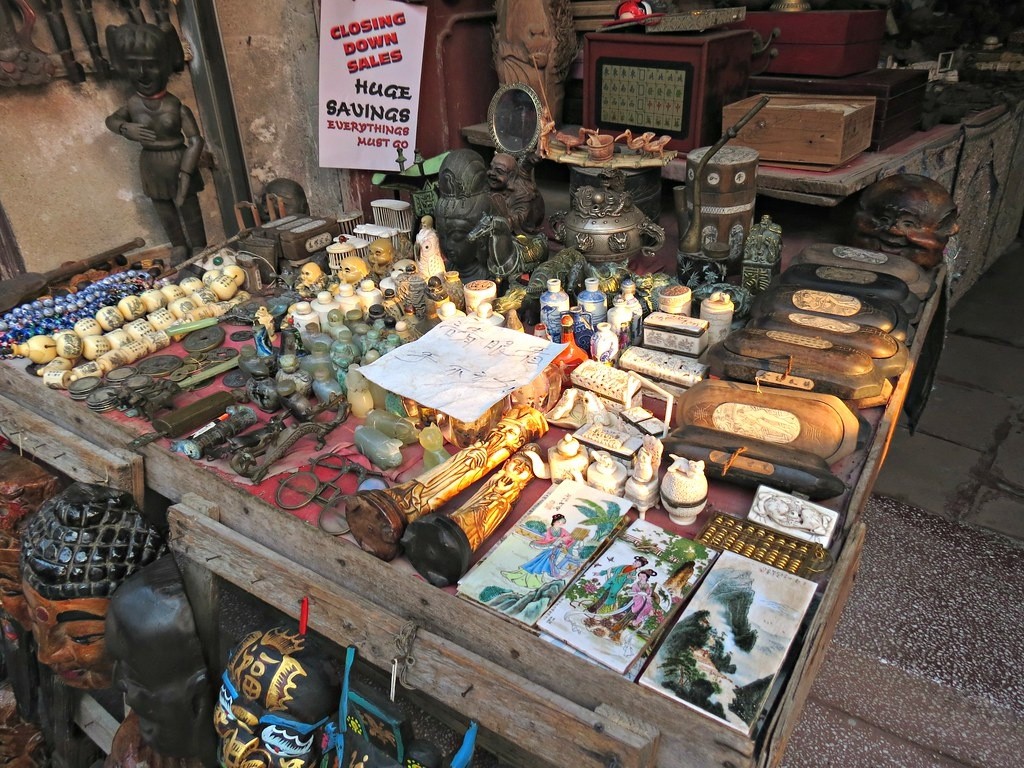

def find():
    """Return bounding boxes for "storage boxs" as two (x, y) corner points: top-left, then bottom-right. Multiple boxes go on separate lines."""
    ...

(257, 213), (342, 261)
(581, 10), (931, 165)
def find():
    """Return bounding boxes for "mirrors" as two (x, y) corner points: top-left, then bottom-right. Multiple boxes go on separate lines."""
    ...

(486, 82), (541, 166)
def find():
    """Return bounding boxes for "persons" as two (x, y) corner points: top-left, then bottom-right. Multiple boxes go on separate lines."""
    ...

(0, 462), (64, 634)
(483, 152), (546, 233)
(296, 233), (416, 300)
(213, 622), (341, 768)
(537, 106), (557, 156)
(105, 551), (219, 759)
(104, 22), (206, 267)
(378, 372), (550, 551)
(414, 215), (445, 279)
(20, 482), (165, 692)
(850, 173), (959, 270)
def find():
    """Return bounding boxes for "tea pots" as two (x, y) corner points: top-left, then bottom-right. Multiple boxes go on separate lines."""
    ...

(569, 448), (634, 498)
(523, 433), (611, 485)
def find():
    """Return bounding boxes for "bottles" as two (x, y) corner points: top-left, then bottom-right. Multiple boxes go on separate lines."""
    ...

(539, 279), (570, 344)
(612, 280), (644, 346)
(577, 277), (606, 332)
(443, 271), (466, 311)
(591, 321), (620, 370)
(535, 323), (552, 343)
(401, 397), (505, 449)
(607, 295), (634, 358)
(549, 316), (589, 401)
(559, 306), (594, 356)
(504, 309), (562, 417)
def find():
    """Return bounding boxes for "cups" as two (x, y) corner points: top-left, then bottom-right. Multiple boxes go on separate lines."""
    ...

(699, 291), (734, 348)
(587, 135), (614, 163)
(658, 286), (691, 317)
